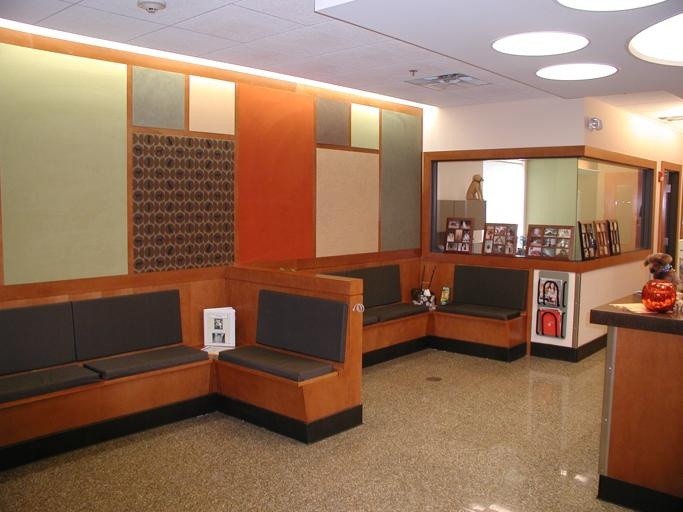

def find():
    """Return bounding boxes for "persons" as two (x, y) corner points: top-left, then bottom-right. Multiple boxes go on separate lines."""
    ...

(212, 333), (225, 346)
(544, 281), (557, 307)
(214, 319), (223, 330)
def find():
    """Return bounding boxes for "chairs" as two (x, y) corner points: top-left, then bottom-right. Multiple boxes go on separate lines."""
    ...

(434, 264), (530, 364)
(217, 275), (365, 450)
(5, 279), (215, 452)
(302, 263), (434, 368)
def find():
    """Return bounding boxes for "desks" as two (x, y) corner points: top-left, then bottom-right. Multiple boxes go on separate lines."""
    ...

(589, 291), (683, 512)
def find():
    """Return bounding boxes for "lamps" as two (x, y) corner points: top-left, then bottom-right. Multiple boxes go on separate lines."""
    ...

(588, 117), (603, 131)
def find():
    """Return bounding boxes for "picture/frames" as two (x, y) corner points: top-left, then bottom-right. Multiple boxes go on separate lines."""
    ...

(525, 223), (576, 261)
(480, 222), (519, 258)
(444, 216), (474, 255)
(577, 219), (621, 261)
